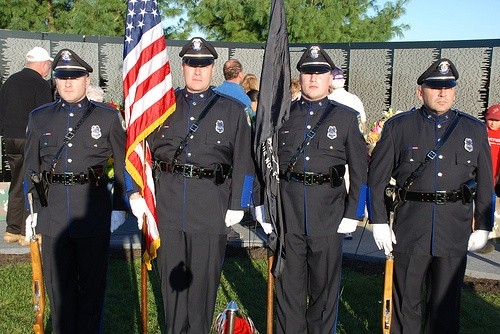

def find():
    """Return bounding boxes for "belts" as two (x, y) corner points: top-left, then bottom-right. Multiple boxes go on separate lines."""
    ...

(396, 189), (460, 204)
(278, 170), (330, 185)
(156, 160), (214, 179)
(43, 172), (87, 186)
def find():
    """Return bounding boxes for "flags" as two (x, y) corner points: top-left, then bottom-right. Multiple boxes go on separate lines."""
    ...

(122, 0), (175, 270)
(254, 0), (292, 275)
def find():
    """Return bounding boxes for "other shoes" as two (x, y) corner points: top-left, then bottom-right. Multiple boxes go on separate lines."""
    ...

(4, 232), (29, 246)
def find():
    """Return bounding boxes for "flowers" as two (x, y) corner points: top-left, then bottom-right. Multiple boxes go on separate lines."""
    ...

(365, 108), (402, 155)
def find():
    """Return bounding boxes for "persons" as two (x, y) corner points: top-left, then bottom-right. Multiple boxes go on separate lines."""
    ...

(125, 37), (255, 334)
(327, 68), (366, 238)
(0, 46), (54, 245)
(25, 49), (126, 334)
(251, 46), (369, 334)
(84, 86), (104, 103)
(369, 58), (493, 334)
(472, 104), (500, 253)
(213, 59), (301, 228)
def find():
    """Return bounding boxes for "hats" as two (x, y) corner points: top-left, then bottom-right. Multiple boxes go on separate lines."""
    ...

(179, 37), (219, 67)
(51, 48), (93, 80)
(331, 67), (344, 80)
(26, 46), (55, 62)
(418, 59), (459, 88)
(297, 43), (335, 74)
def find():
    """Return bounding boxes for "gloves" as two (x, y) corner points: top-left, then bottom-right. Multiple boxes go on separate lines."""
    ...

(467, 229), (490, 252)
(130, 197), (159, 240)
(372, 222), (398, 255)
(256, 205), (274, 235)
(225, 209), (245, 227)
(24, 211), (38, 241)
(336, 217), (359, 234)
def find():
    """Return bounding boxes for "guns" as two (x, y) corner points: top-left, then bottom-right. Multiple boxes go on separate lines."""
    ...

(382, 211), (395, 334)
(27, 192), (46, 334)
(266, 255), (273, 334)
